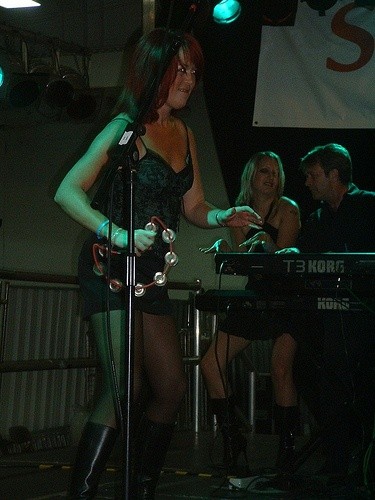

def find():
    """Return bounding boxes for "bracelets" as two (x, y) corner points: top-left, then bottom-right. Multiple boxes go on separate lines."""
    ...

(95, 218), (128, 248)
(215, 209), (226, 227)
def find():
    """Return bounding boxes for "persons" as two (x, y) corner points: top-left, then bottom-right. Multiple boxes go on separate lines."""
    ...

(48, 26), (265, 500)
(283, 141), (373, 488)
(196, 151), (313, 472)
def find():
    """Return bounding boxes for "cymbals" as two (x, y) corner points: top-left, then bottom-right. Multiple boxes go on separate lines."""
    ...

(93, 222), (176, 297)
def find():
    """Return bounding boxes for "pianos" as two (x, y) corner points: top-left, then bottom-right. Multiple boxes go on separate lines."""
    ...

(213, 248), (375, 279)
(194, 289), (375, 341)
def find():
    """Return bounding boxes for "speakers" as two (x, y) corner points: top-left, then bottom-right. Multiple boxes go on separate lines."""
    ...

(167, 0), (209, 47)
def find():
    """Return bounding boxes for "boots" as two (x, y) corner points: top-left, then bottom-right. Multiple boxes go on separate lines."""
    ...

(212, 398), (249, 473)
(133, 417), (173, 500)
(72, 422), (116, 500)
(276, 405), (301, 472)
